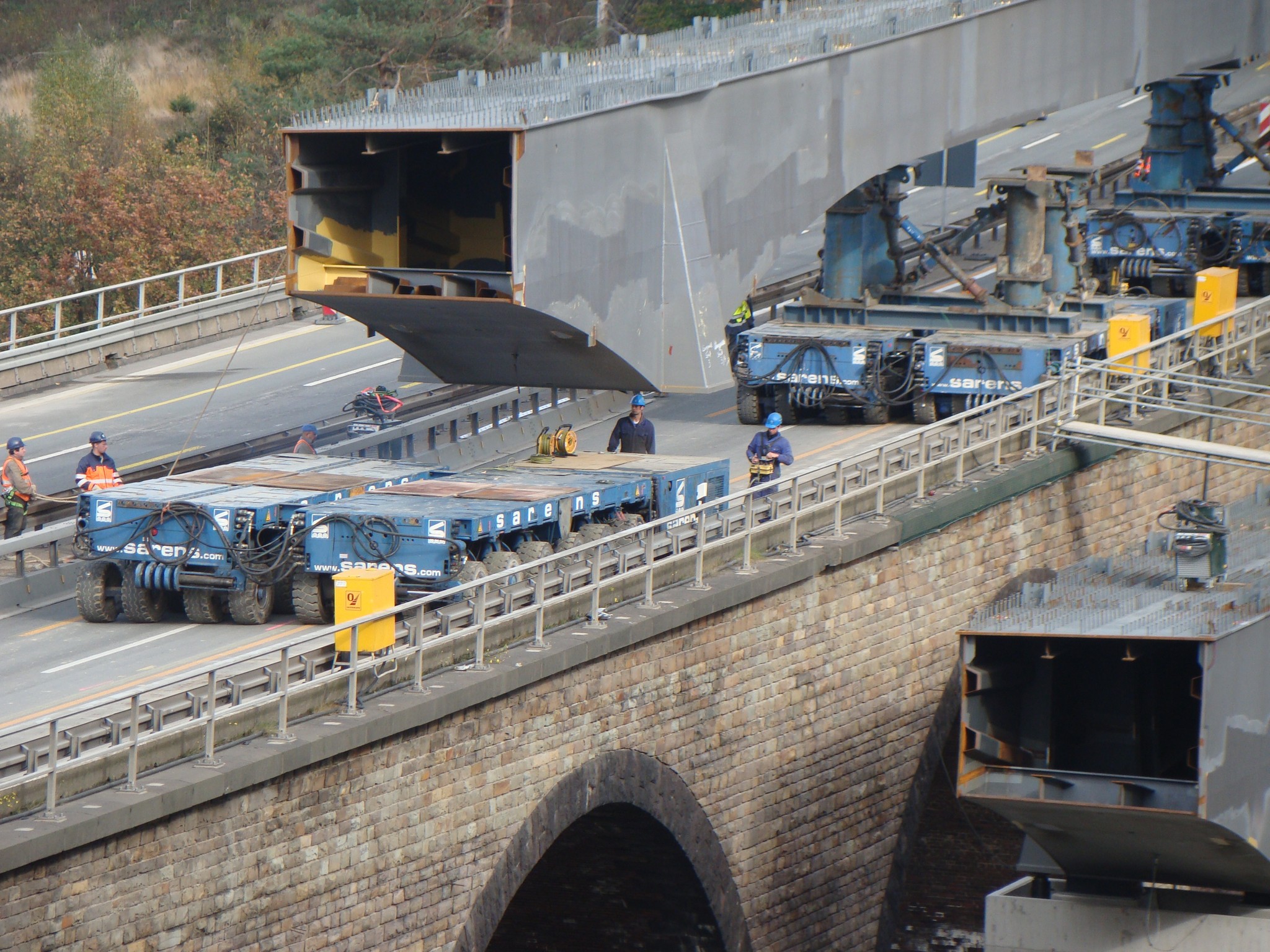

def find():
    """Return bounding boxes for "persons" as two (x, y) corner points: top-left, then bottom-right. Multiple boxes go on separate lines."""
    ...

(746, 413), (794, 523)
(2, 437), (36, 561)
(293, 424), (317, 455)
(607, 395), (655, 454)
(75, 431), (124, 491)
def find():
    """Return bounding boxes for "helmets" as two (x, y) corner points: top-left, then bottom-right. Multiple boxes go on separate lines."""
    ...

(89, 431), (107, 442)
(7, 437), (24, 449)
(302, 425), (317, 433)
(766, 413), (783, 429)
(630, 395), (645, 406)
